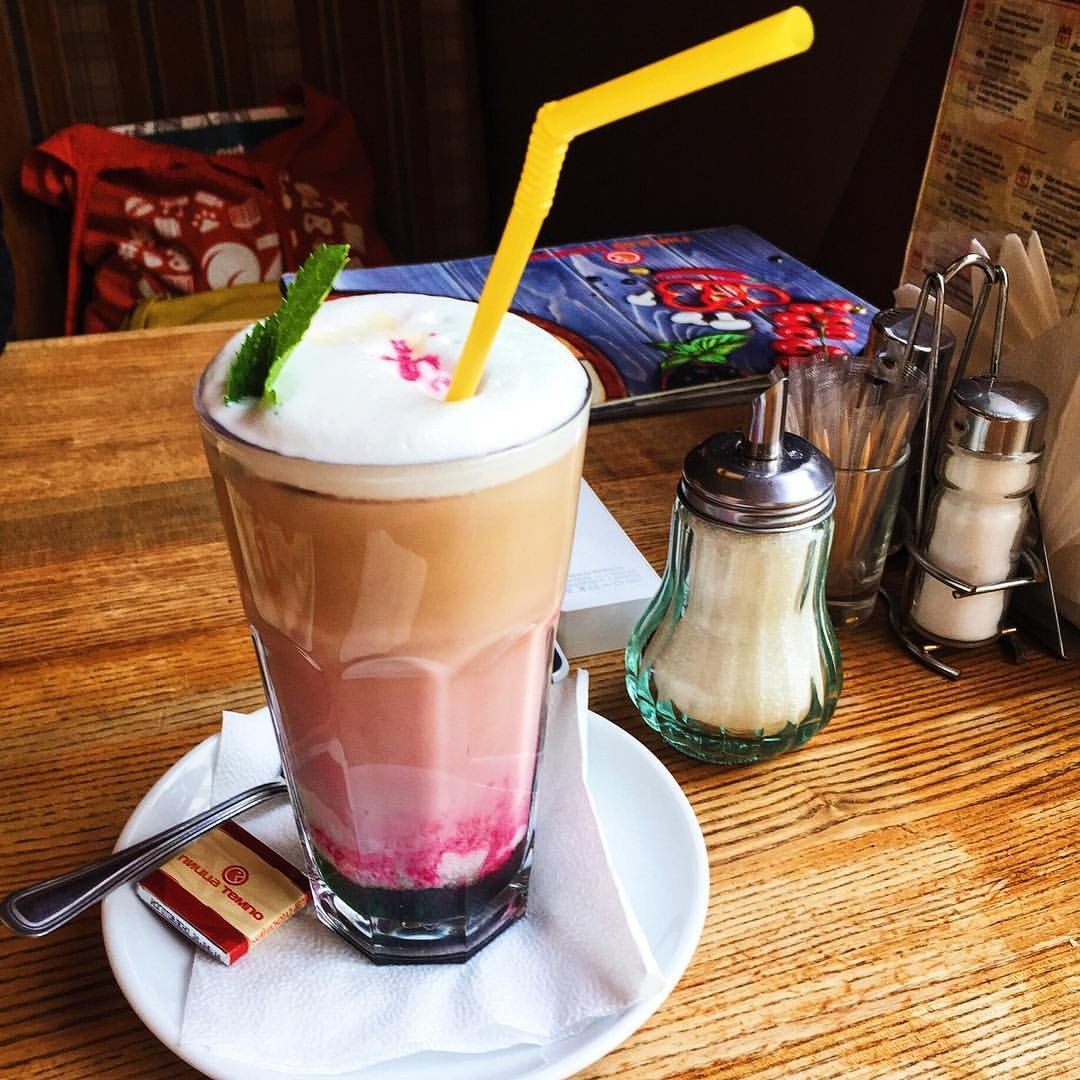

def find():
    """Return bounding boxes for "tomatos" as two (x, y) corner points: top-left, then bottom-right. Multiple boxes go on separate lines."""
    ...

(771, 299), (856, 367)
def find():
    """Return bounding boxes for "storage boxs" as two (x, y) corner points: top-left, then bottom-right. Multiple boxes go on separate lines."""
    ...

(553, 476), (665, 661)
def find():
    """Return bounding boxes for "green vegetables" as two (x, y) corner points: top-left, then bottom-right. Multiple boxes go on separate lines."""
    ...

(642, 333), (754, 369)
(223, 242), (350, 405)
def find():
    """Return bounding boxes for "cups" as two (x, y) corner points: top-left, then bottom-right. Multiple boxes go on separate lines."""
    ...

(195, 292), (594, 971)
(787, 414), (910, 627)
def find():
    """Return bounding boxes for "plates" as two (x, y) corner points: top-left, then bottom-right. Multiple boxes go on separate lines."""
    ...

(100, 697), (711, 1079)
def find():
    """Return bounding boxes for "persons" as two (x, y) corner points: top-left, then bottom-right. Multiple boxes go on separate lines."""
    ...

(0, 197), (18, 358)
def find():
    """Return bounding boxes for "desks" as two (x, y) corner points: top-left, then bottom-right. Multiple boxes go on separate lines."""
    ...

(0, 319), (1080, 1080)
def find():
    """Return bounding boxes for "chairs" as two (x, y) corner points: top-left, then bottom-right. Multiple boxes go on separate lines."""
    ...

(18, 90), (391, 338)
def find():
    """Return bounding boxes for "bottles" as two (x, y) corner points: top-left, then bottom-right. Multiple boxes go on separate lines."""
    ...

(625, 377), (844, 767)
(866, 308), (1050, 650)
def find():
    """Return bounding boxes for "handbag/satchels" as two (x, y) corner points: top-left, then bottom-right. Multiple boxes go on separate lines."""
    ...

(19, 89), (382, 334)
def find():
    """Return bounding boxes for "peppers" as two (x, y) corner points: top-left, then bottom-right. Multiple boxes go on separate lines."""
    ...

(652, 267), (790, 313)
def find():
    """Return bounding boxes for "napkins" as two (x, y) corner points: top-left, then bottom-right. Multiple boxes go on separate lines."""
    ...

(893, 226), (1080, 559)
(179, 665), (661, 1056)
